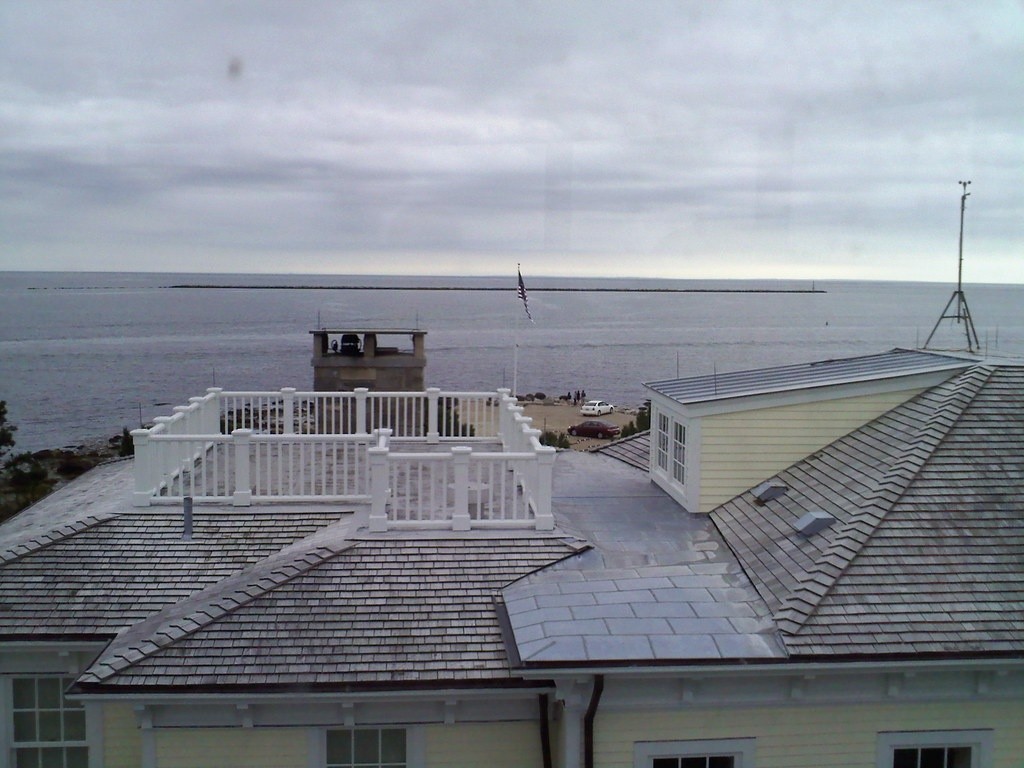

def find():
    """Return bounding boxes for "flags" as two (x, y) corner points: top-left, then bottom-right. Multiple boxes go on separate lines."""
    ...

(518, 272), (532, 319)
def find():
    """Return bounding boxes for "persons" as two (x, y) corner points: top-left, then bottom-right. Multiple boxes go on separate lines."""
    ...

(567, 390), (585, 406)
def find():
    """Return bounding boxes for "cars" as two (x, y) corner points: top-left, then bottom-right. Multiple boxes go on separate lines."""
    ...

(567, 420), (620, 439)
(579, 400), (614, 417)
